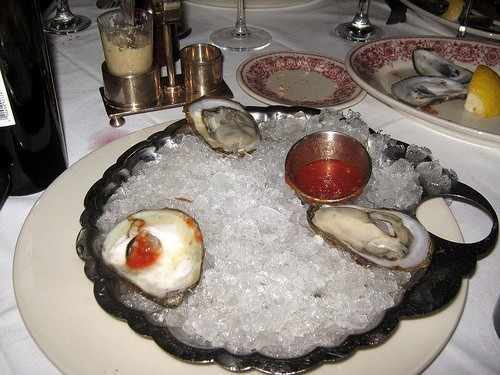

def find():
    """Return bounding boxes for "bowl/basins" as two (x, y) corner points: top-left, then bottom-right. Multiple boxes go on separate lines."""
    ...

(285, 130), (372, 205)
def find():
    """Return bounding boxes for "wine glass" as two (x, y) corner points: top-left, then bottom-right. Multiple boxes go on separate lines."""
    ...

(38, 0), (91, 34)
(209, 0), (273, 52)
(335, 0), (385, 43)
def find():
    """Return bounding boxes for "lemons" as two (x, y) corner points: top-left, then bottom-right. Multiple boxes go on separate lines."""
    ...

(440, 0), (465, 21)
(464, 64), (500, 118)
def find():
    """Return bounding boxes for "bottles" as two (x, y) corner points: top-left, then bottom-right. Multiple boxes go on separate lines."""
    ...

(0, 0), (70, 196)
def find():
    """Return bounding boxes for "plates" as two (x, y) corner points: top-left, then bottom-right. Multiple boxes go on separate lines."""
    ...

(12, 123), (469, 375)
(76, 104), (499, 375)
(180, 0), (320, 12)
(345, 35), (500, 143)
(413, 194), (494, 248)
(396, 0), (500, 42)
(237, 51), (366, 110)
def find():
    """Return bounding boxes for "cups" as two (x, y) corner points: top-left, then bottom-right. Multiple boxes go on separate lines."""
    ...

(101, 62), (163, 111)
(96, 8), (154, 75)
(178, 42), (224, 94)
(160, 0), (184, 33)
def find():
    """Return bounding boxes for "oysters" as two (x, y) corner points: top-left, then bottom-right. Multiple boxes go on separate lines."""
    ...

(183, 95), (263, 158)
(392, 47), (474, 105)
(101, 209), (206, 308)
(307, 203), (434, 272)
